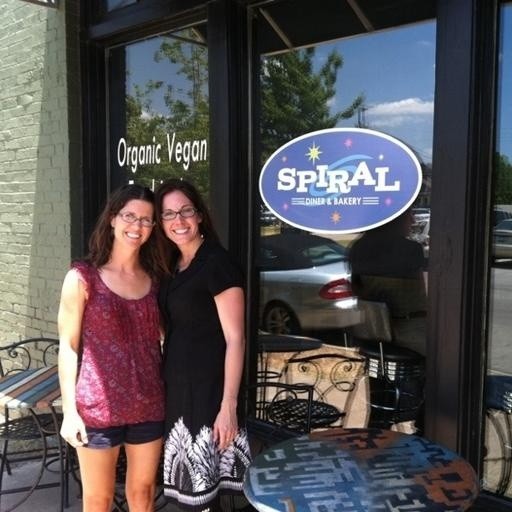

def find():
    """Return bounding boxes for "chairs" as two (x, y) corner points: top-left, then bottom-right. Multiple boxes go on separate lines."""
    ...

(0, 337), (68, 512)
(367, 388), (424, 433)
(222, 382), (314, 512)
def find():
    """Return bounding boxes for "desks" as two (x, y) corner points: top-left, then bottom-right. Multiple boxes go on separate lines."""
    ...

(243, 426), (480, 512)
(0, 366), (71, 512)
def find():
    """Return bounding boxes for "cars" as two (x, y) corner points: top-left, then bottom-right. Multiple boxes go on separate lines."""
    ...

(260, 233), (364, 338)
(408, 214), (430, 262)
(493, 218), (512, 260)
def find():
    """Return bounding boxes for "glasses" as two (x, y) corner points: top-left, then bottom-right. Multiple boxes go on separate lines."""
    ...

(117, 211), (155, 227)
(160, 204), (198, 221)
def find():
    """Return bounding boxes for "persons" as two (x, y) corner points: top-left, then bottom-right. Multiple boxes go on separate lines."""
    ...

(147, 177), (251, 512)
(57, 183), (166, 511)
(343, 203), (429, 339)
